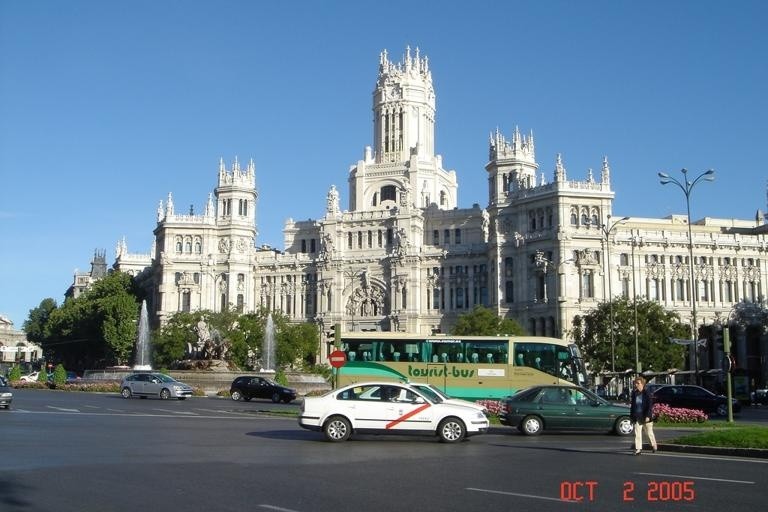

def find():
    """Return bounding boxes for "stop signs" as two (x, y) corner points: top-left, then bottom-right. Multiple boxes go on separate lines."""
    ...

(48, 364), (53, 368)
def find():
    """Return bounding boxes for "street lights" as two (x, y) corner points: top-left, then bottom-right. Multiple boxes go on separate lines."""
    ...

(584, 166), (715, 403)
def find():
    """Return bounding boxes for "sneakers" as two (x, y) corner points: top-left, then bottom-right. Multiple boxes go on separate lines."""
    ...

(633, 448), (657, 457)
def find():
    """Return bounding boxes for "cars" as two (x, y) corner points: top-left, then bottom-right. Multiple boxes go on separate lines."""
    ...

(0, 371), (87, 410)
(230, 376), (298, 405)
(627, 384), (768, 417)
(120, 373), (193, 401)
(499, 384), (640, 438)
(298, 380), (491, 444)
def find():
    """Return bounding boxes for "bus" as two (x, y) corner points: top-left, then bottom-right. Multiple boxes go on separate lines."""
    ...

(592, 367), (732, 403)
(329, 331), (594, 407)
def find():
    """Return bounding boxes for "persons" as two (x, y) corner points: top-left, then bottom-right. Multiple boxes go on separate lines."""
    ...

(629, 376), (658, 457)
(561, 362), (574, 380)
(208, 323), (220, 340)
(197, 316), (209, 342)
(388, 387), (401, 402)
(258, 378), (266, 385)
(565, 391), (577, 406)
